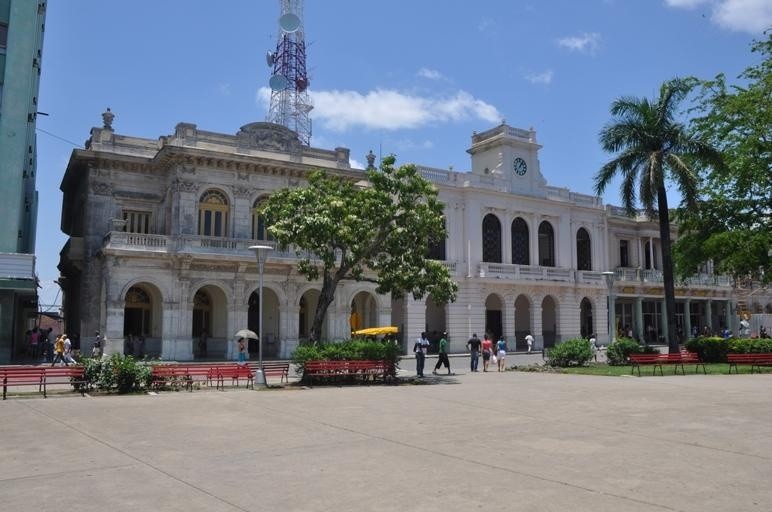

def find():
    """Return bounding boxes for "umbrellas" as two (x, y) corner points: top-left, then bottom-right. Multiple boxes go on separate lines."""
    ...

(235, 329), (259, 340)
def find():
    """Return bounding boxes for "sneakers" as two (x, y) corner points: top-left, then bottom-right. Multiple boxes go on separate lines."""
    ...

(432, 371), (455, 376)
(417, 374), (422, 378)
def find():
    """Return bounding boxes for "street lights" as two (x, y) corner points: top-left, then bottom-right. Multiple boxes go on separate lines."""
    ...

(601, 272), (617, 346)
(248, 245), (273, 388)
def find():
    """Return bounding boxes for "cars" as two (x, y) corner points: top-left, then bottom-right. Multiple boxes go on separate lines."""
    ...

(39, 328), (48, 353)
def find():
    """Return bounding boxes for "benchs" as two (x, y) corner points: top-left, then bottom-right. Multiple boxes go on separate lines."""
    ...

(727, 353), (772, 374)
(628, 352), (707, 375)
(142, 363), (254, 391)
(0, 367), (87, 399)
(304, 360), (396, 387)
(232, 363), (289, 387)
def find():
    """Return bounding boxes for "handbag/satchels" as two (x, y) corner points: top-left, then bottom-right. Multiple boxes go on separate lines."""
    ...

(492, 354), (497, 364)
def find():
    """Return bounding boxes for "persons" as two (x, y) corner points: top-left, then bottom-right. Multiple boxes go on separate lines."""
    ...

(432, 331), (455, 375)
(493, 335), (508, 372)
(198, 329), (207, 356)
(677, 323), (733, 345)
(24, 325), (78, 367)
(413, 331), (430, 378)
(618, 323), (633, 338)
(759, 326), (772, 339)
(92, 343), (100, 360)
(238, 338), (246, 363)
(92, 330), (100, 361)
(589, 334), (597, 351)
(366, 335), (374, 343)
(129, 333), (145, 358)
(381, 334), (390, 345)
(645, 322), (658, 343)
(480, 335), (493, 372)
(466, 333), (482, 372)
(525, 333), (535, 353)
